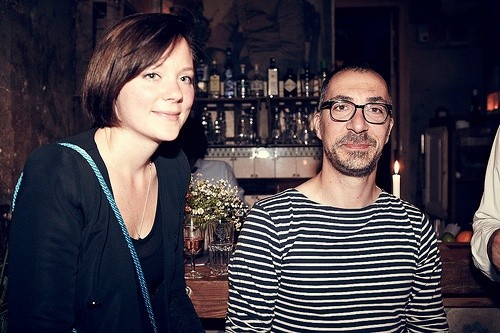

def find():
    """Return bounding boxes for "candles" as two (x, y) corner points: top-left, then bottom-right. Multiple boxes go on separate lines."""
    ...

(392, 159), (401, 200)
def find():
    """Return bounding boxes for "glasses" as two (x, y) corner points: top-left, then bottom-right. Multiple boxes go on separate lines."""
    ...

(313, 99), (393, 125)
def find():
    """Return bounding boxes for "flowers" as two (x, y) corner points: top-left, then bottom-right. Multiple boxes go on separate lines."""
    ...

(184, 172), (249, 232)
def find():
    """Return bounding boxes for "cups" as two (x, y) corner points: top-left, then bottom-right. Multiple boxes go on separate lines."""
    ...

(205, 218), (234, 276)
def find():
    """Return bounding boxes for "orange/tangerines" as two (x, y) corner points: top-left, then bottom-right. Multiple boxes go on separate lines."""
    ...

(442, 232), (455, 242)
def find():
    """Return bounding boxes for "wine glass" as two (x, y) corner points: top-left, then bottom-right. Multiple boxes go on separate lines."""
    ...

(183, 219), (207, 280)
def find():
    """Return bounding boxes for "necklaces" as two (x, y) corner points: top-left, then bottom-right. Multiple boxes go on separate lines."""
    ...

(104, 128), (151, 239)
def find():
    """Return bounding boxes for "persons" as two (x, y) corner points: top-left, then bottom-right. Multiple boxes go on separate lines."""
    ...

(471, 125), (500, 281)
(224, 62), (450, 333)
(0, 13), (206, 333)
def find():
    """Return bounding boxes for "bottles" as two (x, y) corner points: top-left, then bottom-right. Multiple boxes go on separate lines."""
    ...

(469, 89), (481, 127)
(191, 44), (332, 145)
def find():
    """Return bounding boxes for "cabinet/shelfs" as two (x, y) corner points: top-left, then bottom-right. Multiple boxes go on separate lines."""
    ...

(190, 96), (324, 179)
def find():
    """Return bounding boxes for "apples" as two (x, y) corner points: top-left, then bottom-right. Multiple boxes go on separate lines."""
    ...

(456, 231), (473, 242)
